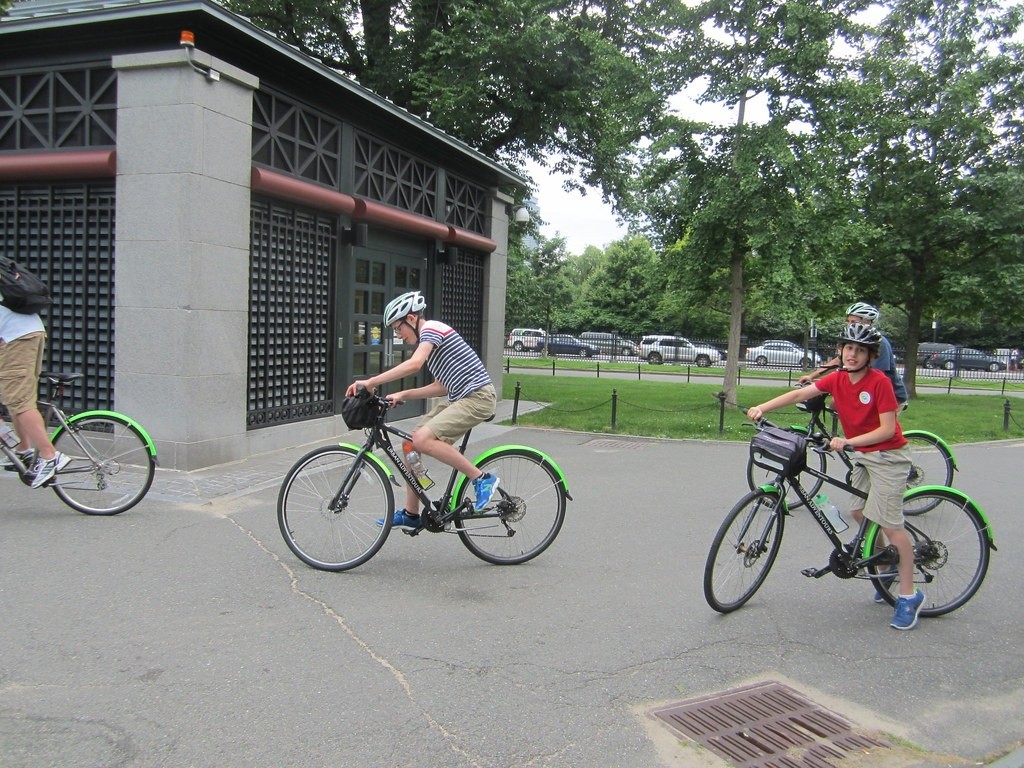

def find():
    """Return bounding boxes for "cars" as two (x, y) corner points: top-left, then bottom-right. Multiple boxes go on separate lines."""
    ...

(579, 332), (639, 356)
(689, 341), (728, 361)
(928, 347), (1007, 373)
(537, 334), (600, 357)
(745, 342), (821, 368)
(761, 339), (818, 353)
(638, 335), (722, 368)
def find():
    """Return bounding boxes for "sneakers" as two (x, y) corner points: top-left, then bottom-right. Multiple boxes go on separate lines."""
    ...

(376, 509), (420, 529)
(890, 588), (925, 630)
(0, 448), (34, 466)
(873, 564), (898, 603)
(31, 451), (72, 489)
(472, 472), (500, 514)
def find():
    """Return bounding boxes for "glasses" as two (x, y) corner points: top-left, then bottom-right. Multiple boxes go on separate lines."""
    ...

(395, 321), (404, 333)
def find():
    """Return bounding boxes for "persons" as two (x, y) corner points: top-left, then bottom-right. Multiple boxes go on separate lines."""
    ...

(345, 291), (500, 531)
(747, 323), (924, 630)
(799, 302), (908, 415)
(1010, 347), (1020, 371)
(0, 294), (71, 489)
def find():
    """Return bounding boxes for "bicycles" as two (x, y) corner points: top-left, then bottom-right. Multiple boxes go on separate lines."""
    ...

(747, 379), (960, 516)
(704, 409), (998, 619)
(278, 383), (574, 571)
(0, 370), (159, 517)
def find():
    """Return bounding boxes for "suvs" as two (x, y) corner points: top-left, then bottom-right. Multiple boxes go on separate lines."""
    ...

(507, 327), (560, 351)
(917, 342), (979, 370)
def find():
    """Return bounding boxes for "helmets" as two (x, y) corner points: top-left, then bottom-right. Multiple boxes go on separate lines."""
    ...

(846, 302), (877, 320)
(839, 322), (883, 351)
(384, 290), (427, 329)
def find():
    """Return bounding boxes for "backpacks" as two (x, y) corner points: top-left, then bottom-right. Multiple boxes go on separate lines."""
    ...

(0, 255), (50, 315)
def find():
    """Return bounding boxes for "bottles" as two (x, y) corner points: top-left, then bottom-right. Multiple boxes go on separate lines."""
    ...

(403, 442), (432, 488)
(814, 494), (848, 532)
(0, 419), (21, 448)
